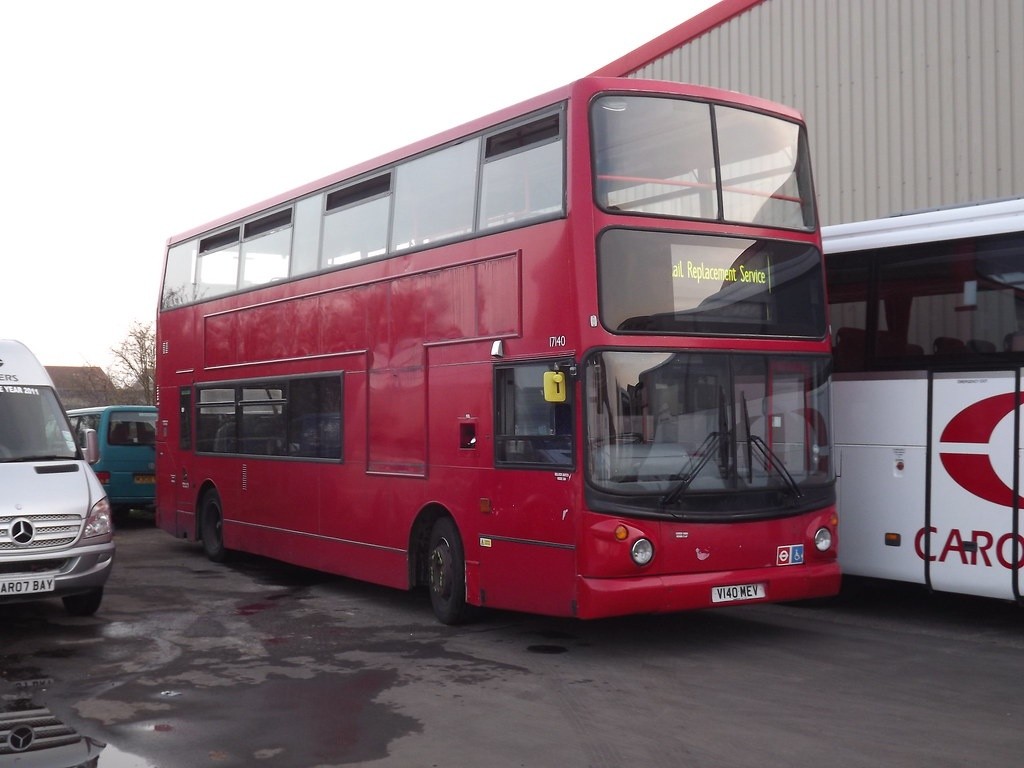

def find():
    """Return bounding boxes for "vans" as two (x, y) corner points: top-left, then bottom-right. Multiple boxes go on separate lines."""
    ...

(0, 337), (114, 617)
(59, 405), (162, 508)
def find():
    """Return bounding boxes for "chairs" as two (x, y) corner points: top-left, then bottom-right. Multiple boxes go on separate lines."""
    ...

(214, 410), (341, 460)
(833, 325), (1024, 362)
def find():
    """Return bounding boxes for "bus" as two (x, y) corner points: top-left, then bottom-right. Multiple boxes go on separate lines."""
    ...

(819, 197), (1024, 604)
(153, 77), (840, 626)
